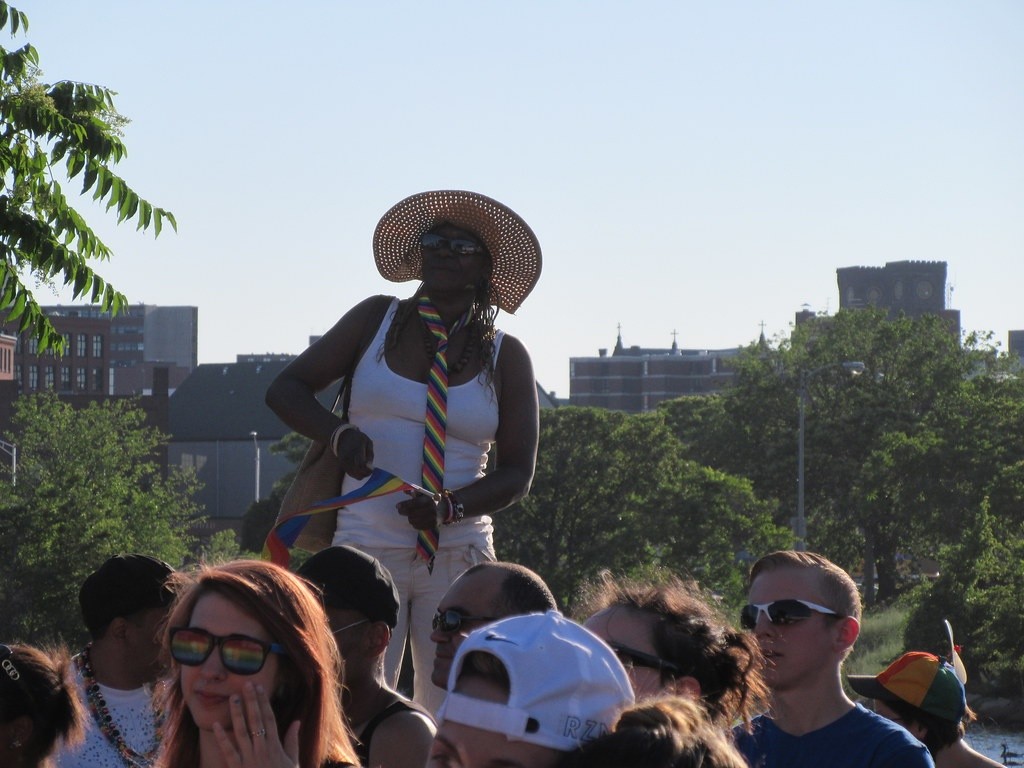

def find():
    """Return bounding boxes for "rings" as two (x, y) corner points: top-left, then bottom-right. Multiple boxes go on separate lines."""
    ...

(250, 728), (266, 737)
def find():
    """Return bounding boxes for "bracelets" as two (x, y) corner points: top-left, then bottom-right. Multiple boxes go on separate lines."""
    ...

(438, 489), (463, 525)
(330, 422), (360, 458)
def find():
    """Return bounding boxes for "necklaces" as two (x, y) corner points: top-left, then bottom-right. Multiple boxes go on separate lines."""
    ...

(416, 302), (477, 375)
(73, 643), (165, 768)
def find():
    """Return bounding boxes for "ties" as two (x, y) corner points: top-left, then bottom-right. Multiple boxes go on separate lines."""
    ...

(402, 297), (476, 574)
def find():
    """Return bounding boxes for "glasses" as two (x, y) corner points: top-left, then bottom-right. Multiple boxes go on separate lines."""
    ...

(421, 232), (485, 257)
(169, 626), (284, 676)
(610, 640), (689, 681)
(431, 610), (497, 634)
(739, 598), (842, 630)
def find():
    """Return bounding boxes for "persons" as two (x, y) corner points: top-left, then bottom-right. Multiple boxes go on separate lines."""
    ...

(729, 551), (935, 768)
(57, 554), (185, 768)
(293, 536), (766, 768)
(266, 189), (544, 723)
(0, 643), (90, 768)
(153, 560), (362, 768)
(847, 651), (1008, 768)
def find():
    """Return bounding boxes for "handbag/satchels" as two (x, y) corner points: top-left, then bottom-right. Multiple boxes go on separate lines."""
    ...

(272, 291), (395, 553)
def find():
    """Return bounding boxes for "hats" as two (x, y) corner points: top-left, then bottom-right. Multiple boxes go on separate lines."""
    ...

(847, 651), (967, 728)
(78, 553), (182, 631)
(434, 609), (636, 753)
(296, 544), (399, 629)
(373, 190), (543, 314)
